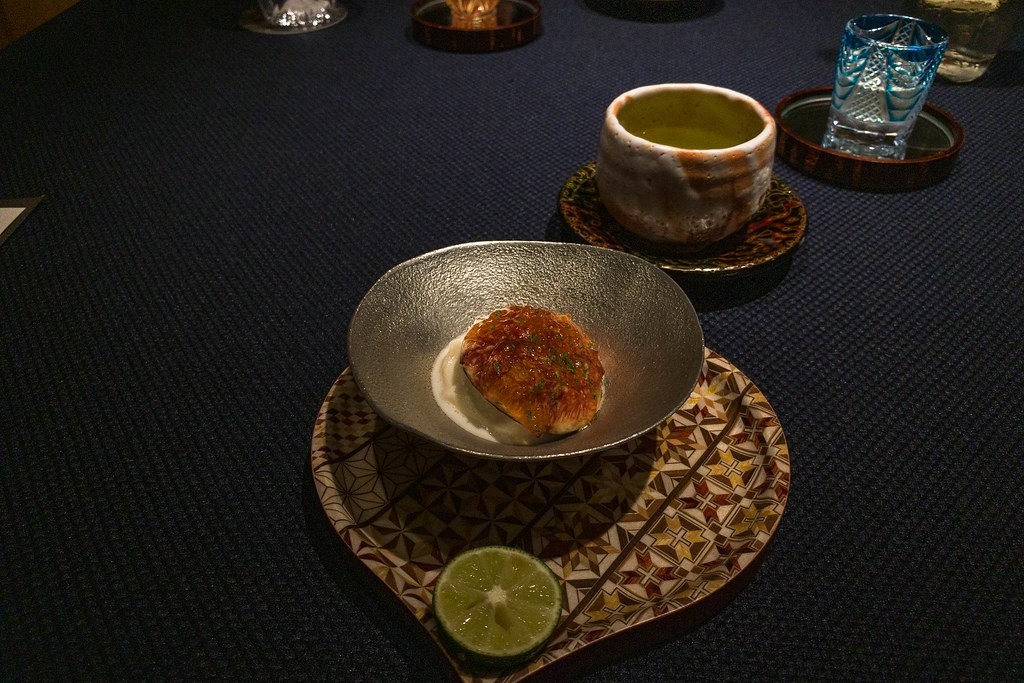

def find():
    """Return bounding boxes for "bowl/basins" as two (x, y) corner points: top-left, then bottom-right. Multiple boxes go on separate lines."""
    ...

(347, 241), (705, 462)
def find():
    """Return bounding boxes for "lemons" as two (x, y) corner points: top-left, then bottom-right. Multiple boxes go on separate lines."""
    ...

(434, 548), (563, 657)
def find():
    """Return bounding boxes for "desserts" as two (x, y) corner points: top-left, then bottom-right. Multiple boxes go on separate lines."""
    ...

(461, 307), (606, 439)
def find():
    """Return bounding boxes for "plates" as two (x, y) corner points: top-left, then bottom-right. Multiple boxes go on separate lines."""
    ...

(560, 161), (804, 275)
(308, 348), (790, 683)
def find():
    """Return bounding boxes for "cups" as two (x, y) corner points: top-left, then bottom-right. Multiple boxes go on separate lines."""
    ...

(256, 0), (335, 27)
(448, 0), (498, 19)
(901, 0), (1021, 83)
(595, 85), (776, 242)
(451, 17), (497, 28)
(827, 15), (949, 143)
(822, 126), (907, 157)
